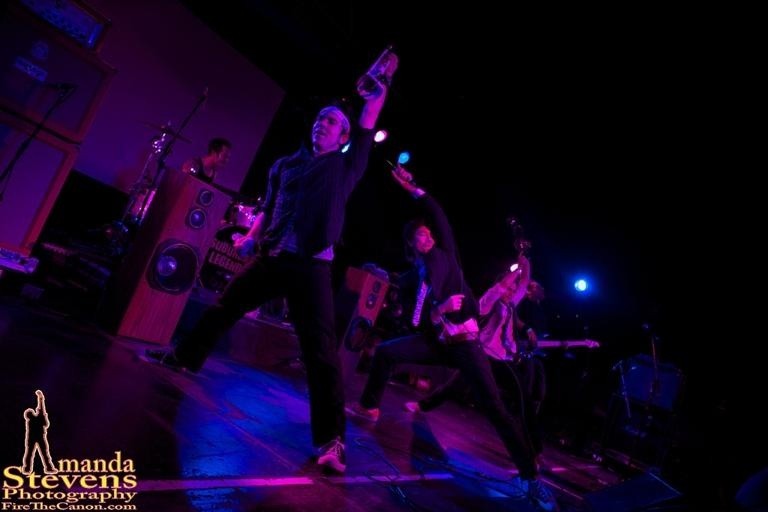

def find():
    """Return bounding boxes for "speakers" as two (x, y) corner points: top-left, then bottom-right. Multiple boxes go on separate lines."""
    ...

(0, 0), (119, 275)
(584, 473), (689, 512)
(333, 266), (391, 386)
(115, 166), (233, 347)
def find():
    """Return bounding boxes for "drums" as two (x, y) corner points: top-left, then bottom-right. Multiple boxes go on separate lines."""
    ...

(199, 223), (261, 296)
(228, 201), (261, 230)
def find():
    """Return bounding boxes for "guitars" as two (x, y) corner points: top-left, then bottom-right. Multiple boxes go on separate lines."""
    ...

(517, 333), (601, 362)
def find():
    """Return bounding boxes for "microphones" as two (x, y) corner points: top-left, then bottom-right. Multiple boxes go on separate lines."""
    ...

(32, 79), (76, 90)
(612, 360), (622, 370)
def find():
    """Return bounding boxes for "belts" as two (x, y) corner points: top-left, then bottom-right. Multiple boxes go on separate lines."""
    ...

(440, 333), (477, 345)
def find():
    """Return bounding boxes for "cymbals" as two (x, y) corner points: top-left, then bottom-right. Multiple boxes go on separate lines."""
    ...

(139, 117), (191, 144)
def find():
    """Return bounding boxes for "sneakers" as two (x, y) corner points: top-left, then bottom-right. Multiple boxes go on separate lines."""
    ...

(528, 481), (556, 512)
(344, 402), (379, 422)
(316, 440), (348, 474)
(142, 347), (183, 370)
(405, 401), (425, 414)
(537, 460), (550, 474)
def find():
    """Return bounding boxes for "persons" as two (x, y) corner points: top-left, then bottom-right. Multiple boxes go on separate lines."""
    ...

(343, 161), (569, 511)
(140, 52), (399, 477)
(180, 136), (232, 186)
